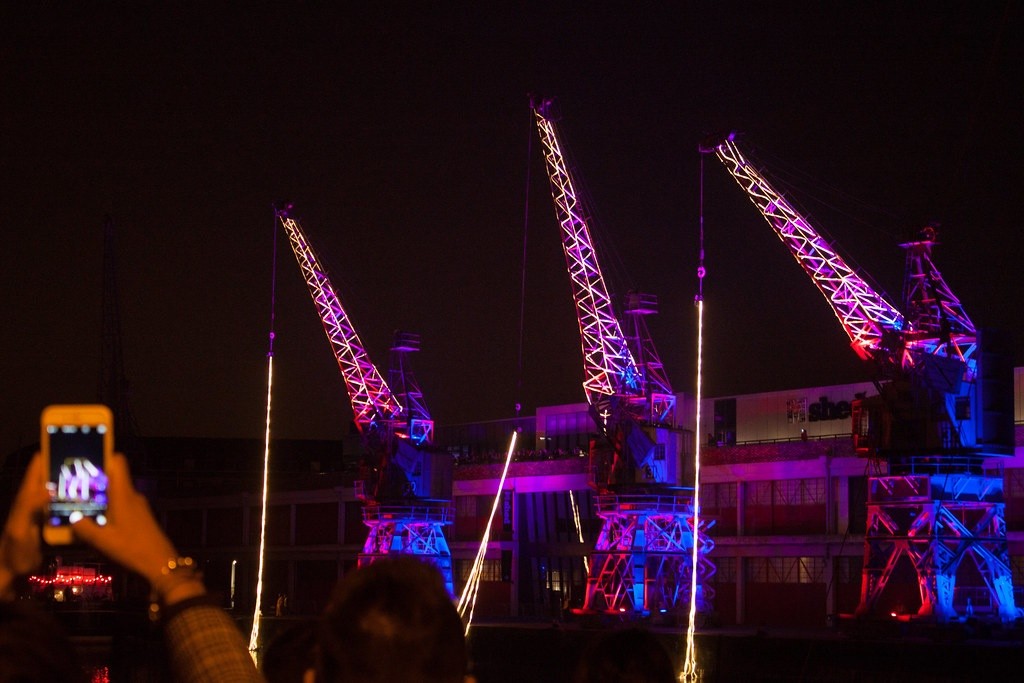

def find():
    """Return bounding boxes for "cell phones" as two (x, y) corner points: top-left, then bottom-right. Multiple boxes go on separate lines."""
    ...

(39, 405), (112, 545)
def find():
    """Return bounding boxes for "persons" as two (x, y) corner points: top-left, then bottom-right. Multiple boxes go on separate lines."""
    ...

(0, 448), (265, 683)
(464, 445), (581, 462)
(275, 592), (289, 616)
(301, 555), (677, 683)
(261, 617), (324, 683)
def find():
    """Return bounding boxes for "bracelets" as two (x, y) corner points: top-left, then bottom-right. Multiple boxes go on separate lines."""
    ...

(148, 555), (220, 623)
